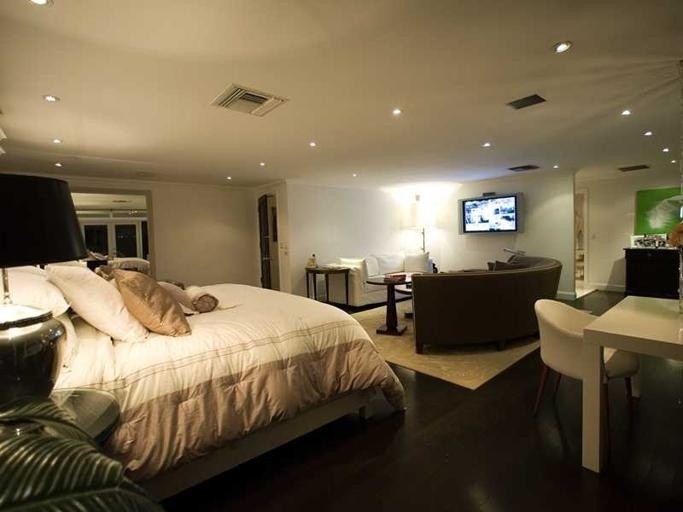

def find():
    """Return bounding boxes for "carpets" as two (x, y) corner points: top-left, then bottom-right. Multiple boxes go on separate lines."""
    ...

(349, 299), (592, 391)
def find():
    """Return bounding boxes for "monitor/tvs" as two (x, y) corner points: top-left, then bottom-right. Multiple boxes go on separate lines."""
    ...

(458, 192), (525, 235)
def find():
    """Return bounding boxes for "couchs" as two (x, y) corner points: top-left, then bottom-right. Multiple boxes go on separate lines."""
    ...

(323, 257), (433, 308)
(411, 255), (563, 354)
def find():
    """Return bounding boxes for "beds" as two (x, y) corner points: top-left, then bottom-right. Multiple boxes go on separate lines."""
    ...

(50, 283), (406, 503)
(107, 257), (151, 275)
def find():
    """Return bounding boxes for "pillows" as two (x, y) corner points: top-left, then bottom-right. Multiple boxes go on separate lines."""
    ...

(6, 261), (218, 341)
(336, 252), (429, 277)
(487, 261), (528, 270)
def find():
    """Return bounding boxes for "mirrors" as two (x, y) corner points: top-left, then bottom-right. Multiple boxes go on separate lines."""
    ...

(70, 186), (157, 284)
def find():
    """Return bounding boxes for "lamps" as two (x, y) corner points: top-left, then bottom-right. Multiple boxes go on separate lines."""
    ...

(0, 172), (88, 400)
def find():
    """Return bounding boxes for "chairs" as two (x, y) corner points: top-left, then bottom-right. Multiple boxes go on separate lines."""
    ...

(532, 299), (640, 447)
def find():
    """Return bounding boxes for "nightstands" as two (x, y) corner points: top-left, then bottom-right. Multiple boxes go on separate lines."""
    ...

(47, 388), (120, 447)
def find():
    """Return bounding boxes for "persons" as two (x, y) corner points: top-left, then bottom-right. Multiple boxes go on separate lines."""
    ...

(466, 198), (515, 231)
(670, 222), (683, 314)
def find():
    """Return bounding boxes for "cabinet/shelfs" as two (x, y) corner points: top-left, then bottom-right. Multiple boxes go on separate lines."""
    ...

(623, 247), (680, 299)
